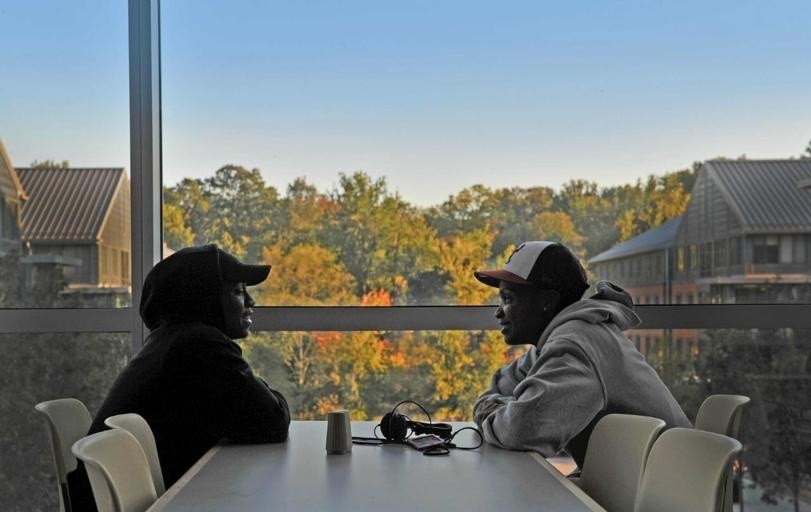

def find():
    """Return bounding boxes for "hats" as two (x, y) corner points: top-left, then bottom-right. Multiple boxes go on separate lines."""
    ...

(473, 240), (590, 290)
(218, 248), (271, 286)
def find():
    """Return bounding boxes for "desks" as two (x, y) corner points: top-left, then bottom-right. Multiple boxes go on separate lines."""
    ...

(142, 419), (606, 510)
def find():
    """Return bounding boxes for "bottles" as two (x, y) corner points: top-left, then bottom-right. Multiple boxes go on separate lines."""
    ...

(325, 410), (353, 456)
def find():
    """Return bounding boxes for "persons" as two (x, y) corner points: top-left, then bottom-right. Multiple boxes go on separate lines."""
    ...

(68, 244), (290, 511)
(472, 241), (695, 485)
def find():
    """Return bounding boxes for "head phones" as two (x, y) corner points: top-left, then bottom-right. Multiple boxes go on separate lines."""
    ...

(380, 412), (452, 441)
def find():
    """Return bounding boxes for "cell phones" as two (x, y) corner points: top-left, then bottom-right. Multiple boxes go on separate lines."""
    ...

(407, 433), (446, 450)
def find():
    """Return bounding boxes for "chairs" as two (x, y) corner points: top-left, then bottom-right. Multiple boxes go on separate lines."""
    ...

(33, 396), (165, 512)
(577, 388), (756, 512)
